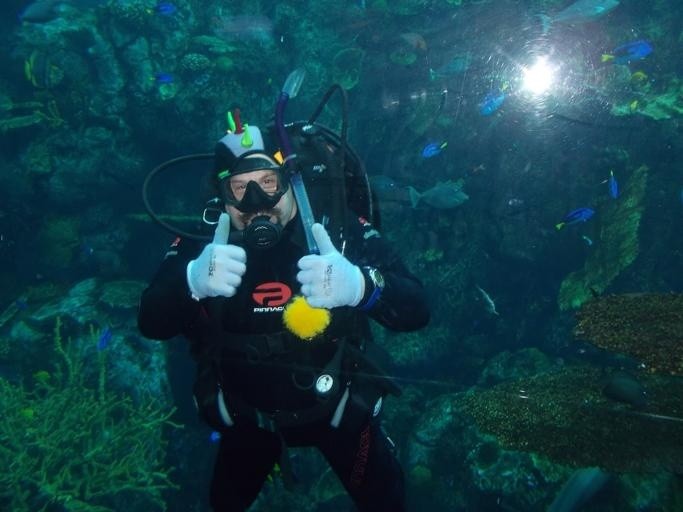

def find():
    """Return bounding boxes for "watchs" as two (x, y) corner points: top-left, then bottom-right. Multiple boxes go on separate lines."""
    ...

(357, 265), (385, 311)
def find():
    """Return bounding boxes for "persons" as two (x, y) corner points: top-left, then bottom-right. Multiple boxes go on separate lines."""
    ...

(138, 83), (430, 512)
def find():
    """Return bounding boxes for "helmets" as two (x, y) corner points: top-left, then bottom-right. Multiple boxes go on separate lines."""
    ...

(213, 124), (288, 185)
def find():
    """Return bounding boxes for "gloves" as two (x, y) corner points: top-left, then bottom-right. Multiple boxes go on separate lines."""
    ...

(295, 222), (365, 310)
(185, 211), (249, 300)
